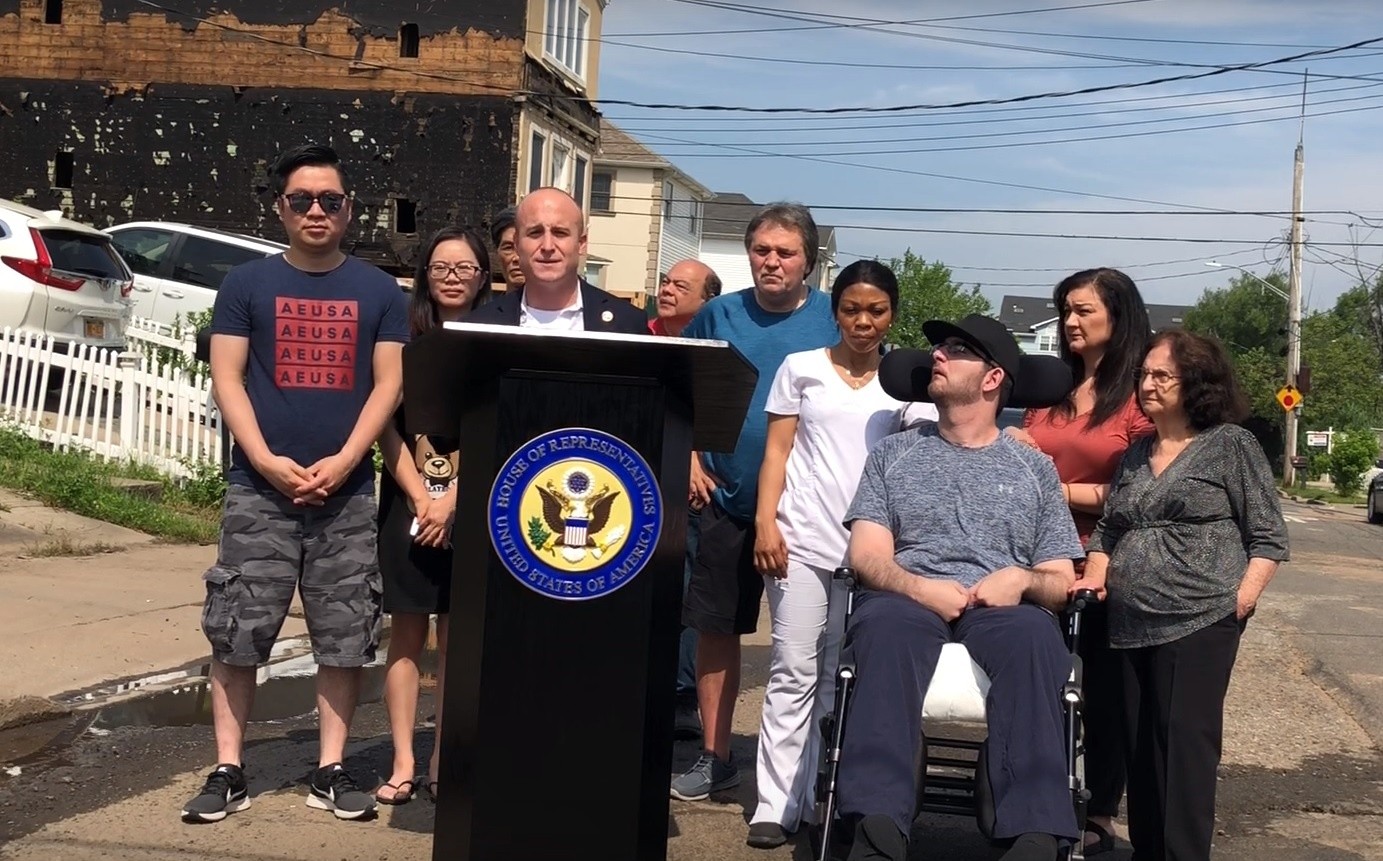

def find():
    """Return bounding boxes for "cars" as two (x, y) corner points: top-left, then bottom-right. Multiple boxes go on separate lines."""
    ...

(1366, 458), (1383, 525)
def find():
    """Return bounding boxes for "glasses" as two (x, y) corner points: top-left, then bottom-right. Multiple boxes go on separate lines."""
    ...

(424, 263), (484, 282)
(1132, 367), (1182, 384)
(281, 192), (348, 214)
(935, 340), (999, 368)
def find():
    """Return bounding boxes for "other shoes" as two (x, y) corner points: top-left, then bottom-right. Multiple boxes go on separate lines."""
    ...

(747, 822), (789, 845)
(674, 704), (702, 735)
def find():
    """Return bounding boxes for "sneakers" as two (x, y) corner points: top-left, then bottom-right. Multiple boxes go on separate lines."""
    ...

(305, 762), (379, 819)
(671, 749), (742, 801)
(181, 762), (252, 821)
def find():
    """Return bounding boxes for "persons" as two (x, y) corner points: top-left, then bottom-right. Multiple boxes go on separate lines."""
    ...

(377, 186), (1153, 861)
(182, 144), (408, 823)
(1068, 328), (1290, 861)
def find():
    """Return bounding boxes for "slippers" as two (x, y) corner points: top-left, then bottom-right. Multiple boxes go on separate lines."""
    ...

(427, 781), (437, 803)
(376, 780), (415, 805)
(1074, 819), (1118, 856)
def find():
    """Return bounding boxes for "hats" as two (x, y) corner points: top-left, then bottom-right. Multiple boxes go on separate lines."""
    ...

(922, 312), (1021, 395)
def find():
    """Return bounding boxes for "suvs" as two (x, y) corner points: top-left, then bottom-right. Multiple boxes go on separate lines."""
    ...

(99, 220), (291, 344)
(1, 197), (135, 401)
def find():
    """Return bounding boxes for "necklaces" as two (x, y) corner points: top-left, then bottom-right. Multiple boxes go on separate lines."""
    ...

(852, 381), (859, 389)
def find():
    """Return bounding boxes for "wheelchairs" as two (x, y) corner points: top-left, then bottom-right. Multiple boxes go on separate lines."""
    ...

(805, 567), (1095, 861)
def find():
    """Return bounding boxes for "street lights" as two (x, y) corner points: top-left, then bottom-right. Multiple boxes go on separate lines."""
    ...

(1205, 260), (1302, 489)
